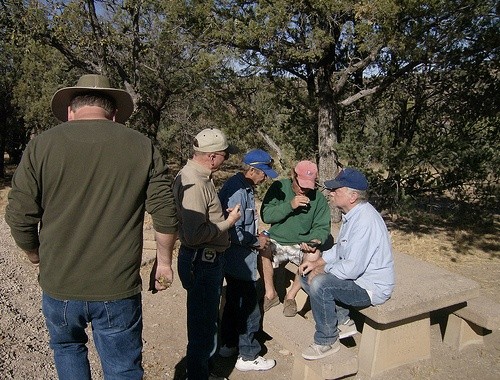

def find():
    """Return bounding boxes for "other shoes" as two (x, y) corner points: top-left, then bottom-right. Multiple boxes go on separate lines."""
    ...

(283, 295), (297, 317)
(263, 295), (279, 312)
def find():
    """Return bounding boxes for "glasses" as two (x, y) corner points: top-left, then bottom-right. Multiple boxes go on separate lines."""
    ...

(249, 159), (274, 168)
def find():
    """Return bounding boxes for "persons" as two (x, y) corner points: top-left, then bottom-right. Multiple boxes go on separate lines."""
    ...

(173, 127), (241, 378)
(297, 167), (395, 359)
(221, 149), (277, 371)
(4, 75), (177, 380)
(261, 161), (331, 316)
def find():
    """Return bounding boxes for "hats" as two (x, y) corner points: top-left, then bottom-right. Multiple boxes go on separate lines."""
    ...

(192, 128), (239, 154)
(295, 161), (317, 191)
(243, 148), (278, 178)
(51, 75), (133, 123)
(324, 167), (369, 190)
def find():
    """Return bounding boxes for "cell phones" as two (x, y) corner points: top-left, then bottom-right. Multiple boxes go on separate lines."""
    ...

(304, 242), (319, 247)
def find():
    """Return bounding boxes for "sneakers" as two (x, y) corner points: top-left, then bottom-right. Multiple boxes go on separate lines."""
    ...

(337, 319), (357, 339)
(302, 338), (340, 360)
(235, 355), (276, 371)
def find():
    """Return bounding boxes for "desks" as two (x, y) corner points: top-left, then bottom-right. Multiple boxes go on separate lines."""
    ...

(283, 244), (481, 377)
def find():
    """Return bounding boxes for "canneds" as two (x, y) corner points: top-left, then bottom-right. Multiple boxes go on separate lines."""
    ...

(262, 230), (270, 236)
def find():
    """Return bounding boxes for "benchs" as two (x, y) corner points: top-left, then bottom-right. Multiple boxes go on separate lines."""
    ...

(262, 302), (360, 380)
(441, 298), (500, 348)
